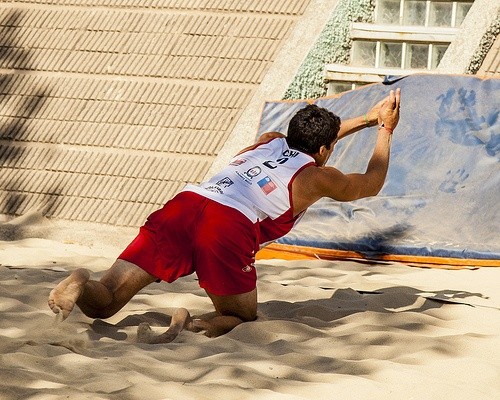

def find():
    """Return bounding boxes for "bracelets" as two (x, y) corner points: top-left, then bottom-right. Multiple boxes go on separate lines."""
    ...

(379, 126), (393, 134)
(364, 114), (369, 127)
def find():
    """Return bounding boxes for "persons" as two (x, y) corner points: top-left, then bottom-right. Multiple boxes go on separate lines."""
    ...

(49, 86), (403, 336)
